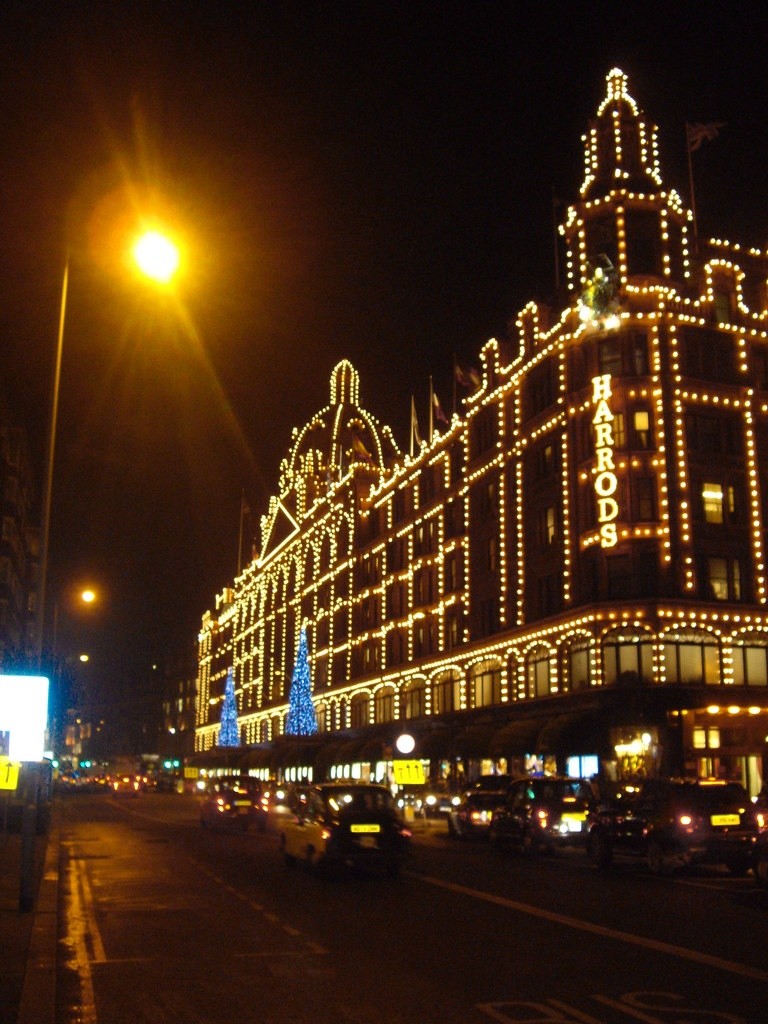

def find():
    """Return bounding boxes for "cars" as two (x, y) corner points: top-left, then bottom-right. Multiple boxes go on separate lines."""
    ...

(584, 778), (761, 872)
(393, 775), (518, 840)
(487, 777), (596, 854)
(262, 781), (290, 808)
(199, 776), (271, 831)
(276, 781), (413, 875)
(90, 771), (174, 798)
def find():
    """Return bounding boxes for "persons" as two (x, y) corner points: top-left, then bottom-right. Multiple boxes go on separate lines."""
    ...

(589, 778), (600, 801)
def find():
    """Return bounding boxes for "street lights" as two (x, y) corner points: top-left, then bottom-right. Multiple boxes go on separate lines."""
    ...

(33, 169), (188, 672)
(54, 649), (88, 753)
(49, 583), (98, 757)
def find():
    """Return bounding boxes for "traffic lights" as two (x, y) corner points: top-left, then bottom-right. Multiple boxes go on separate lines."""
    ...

(81, 761), (92, 768)
(164, 760), (179, 769)
(51, 760), (60, 768)
